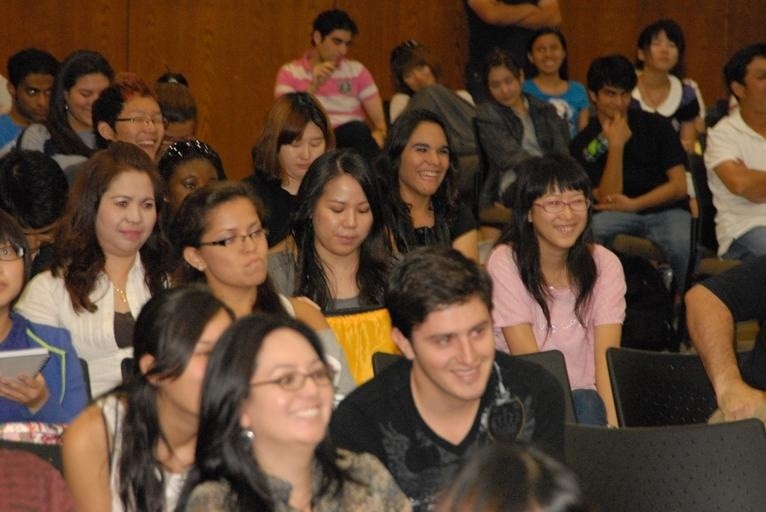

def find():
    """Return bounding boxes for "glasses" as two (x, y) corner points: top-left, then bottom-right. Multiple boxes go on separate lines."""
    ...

(0, 244), (24, 261)
(533, 199), (590, 213)
(199, 229), (270, 247)
(250, 365), (337, 391)
(115, 117), (167, 127)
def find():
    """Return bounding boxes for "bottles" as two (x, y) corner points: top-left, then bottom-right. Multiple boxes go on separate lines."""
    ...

(583, 131), (610, 164)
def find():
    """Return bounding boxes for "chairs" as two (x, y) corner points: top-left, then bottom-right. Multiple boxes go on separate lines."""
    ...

(0, 84), (765, 512)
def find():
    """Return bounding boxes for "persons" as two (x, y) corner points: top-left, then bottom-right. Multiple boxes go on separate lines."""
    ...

(436, 440), (584, 512)
(2, 26), (766, 450)
(464, 0), (562, 101)
(334, 246), (564, 511)
(63, 287), (237, 512)
(273, 8), (387, 155)
(174, 313), (412, 512)
